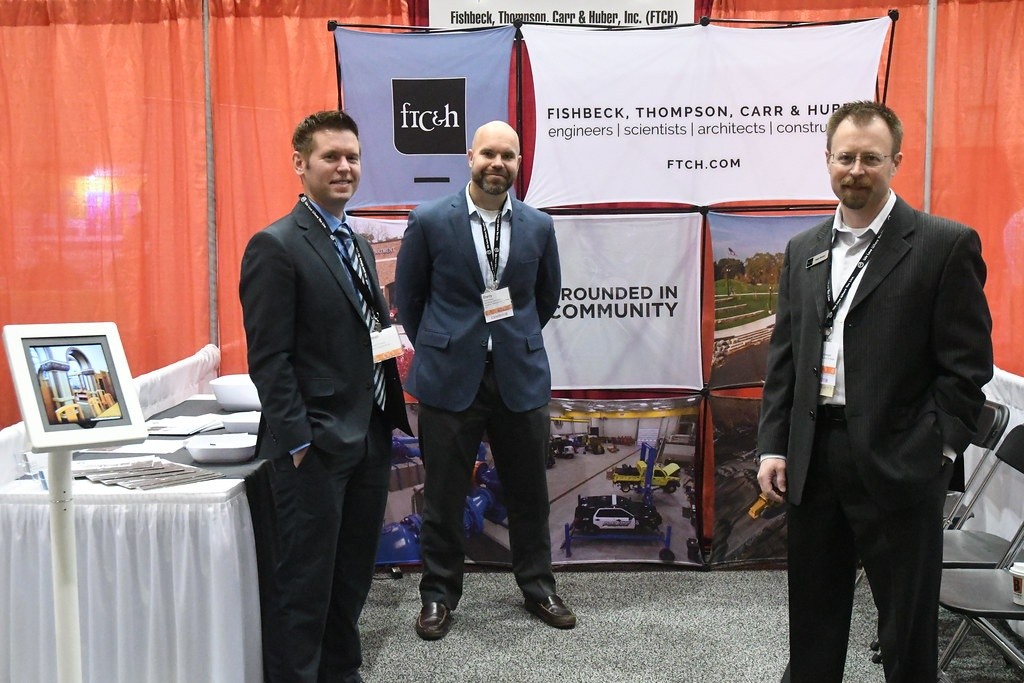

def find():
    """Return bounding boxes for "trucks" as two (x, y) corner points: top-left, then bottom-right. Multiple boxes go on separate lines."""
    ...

(606, 460), (681, 493)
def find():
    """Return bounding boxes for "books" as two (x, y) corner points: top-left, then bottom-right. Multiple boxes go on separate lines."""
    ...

(77, 439), (185, 452)
(146, 411), (261, 434)
(71, 453), (226, 490)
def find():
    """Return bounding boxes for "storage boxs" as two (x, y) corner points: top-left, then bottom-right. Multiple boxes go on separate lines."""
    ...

(389, 457), (425, 492)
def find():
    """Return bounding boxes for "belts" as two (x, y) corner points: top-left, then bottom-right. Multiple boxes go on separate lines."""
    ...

(815, 404), (848, 426)
(483, 351), (494, 367)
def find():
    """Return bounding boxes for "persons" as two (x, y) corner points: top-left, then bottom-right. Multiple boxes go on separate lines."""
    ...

(240, 110), (414, 683)
(756, 100), (994, 681)
(395, 120), (577, 639)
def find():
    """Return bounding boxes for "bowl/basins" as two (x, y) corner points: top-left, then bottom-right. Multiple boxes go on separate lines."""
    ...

(208, 373), (262, 411)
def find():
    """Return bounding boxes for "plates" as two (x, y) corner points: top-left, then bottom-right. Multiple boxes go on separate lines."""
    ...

(186, 435), (257, 463)
(223, 411), (260, 433)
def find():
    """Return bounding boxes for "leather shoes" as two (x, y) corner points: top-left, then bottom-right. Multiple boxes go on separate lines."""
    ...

(416, 599), (451, 638)
(525, 591), (576, 630)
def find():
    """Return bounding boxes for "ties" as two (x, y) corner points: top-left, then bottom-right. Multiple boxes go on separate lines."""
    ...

(338, 224), (387, 413)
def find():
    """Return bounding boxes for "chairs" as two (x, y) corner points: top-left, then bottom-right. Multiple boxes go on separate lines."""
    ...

(872, 424), (1024, 672)
(937, 537), (1024, 683)
(854, 400), (1009, 652)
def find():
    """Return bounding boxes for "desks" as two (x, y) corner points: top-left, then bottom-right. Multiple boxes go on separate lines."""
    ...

(0, 344), (268, 683)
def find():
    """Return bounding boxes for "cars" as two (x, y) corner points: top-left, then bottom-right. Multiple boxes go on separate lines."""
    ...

(587, 508), (649, 537)
